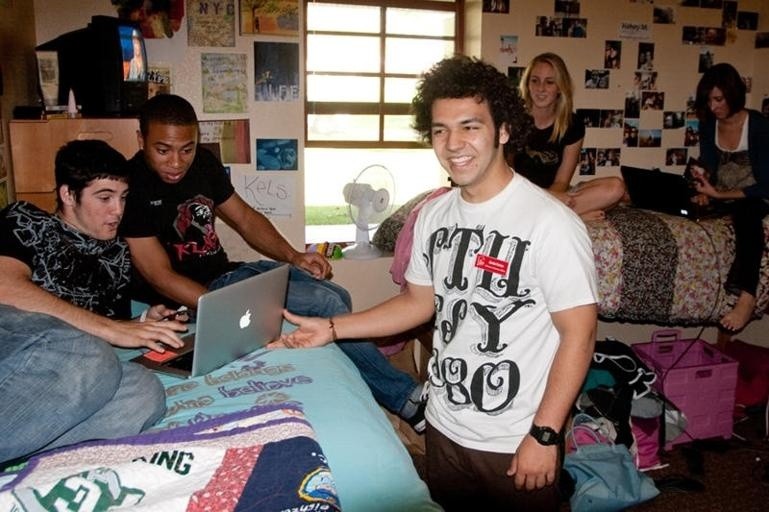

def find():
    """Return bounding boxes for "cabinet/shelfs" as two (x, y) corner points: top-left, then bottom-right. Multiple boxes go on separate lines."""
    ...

(8, 117), (141, 213)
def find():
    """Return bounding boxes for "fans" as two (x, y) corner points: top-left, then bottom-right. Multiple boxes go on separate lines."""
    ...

(341, 164), (396, 260)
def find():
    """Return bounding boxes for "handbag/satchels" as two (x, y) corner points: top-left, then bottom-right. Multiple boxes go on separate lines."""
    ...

(562, 413), (661, 512)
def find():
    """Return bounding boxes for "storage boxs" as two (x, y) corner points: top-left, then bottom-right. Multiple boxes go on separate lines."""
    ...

(632, 330), (740, 451)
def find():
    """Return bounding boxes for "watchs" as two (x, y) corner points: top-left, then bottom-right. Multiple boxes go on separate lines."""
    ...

(529, 423), (560, 446)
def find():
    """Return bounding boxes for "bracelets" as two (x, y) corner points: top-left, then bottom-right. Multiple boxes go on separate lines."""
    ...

(328, 315), (339, 341)
(139, 309), (148, 323)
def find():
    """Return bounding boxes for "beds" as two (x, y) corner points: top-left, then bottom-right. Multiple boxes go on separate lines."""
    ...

(373, 190), (769, 342)
(0, 299), (443, 512)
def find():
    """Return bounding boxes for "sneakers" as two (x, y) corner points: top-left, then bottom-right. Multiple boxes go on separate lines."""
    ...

(407, 380), (429, 432)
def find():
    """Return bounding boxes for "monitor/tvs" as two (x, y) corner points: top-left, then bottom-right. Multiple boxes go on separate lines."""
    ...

(34, 14), (148, 116)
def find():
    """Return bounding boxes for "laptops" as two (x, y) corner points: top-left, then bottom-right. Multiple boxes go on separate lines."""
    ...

(127, 261), (290, 379)
(620, 165), (725, 219)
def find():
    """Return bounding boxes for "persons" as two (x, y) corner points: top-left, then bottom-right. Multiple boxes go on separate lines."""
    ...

(0, 139), (189, 464)
(266, 53), (600, 512)
(117, 93), (429, 433)
(504, 54), (626, 220)
(537, 2), (768, 176)
(688, 63), (769, 332)
(129, 30), (147, 83)
